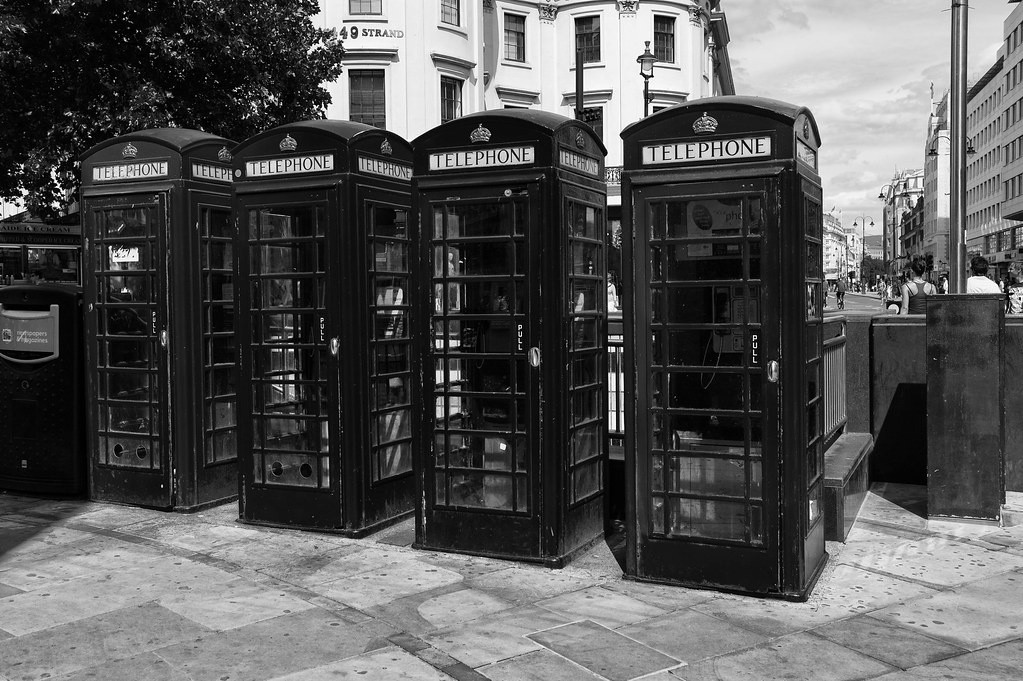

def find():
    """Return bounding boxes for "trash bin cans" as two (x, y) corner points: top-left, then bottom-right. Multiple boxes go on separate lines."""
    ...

(0, 286), (90, 498)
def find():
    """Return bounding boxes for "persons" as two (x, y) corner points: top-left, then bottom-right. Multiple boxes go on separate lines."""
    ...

(900, 260), (936, 314)
(834, 278), (845, 305)
(939, 276), (948, 294)
(967, 257), (1002, 294)
(999, 277), (1014, 314)
(877, 278), (900, 305)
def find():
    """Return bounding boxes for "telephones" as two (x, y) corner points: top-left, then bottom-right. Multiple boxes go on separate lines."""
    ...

(711, 284), (761, 355)
(475, 279), (524, 330)
